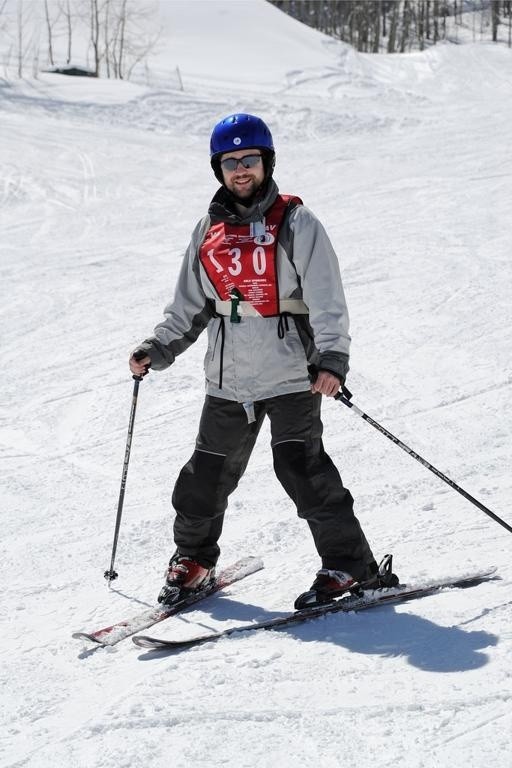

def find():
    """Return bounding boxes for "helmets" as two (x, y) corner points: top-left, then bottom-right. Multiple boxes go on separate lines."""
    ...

(210, 114), (274, 157)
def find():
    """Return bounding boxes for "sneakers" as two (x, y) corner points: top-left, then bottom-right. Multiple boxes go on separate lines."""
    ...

(310, 568), (362, 601)
(165, 551), (216, 591)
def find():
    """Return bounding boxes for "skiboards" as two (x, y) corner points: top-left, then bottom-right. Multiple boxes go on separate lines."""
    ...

(71, 555), (497, 649)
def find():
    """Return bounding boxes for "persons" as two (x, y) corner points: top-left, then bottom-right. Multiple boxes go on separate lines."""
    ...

(128, 113), (382, 609)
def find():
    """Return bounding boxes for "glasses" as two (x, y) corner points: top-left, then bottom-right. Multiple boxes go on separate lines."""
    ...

(221, 155), (262, 170)
